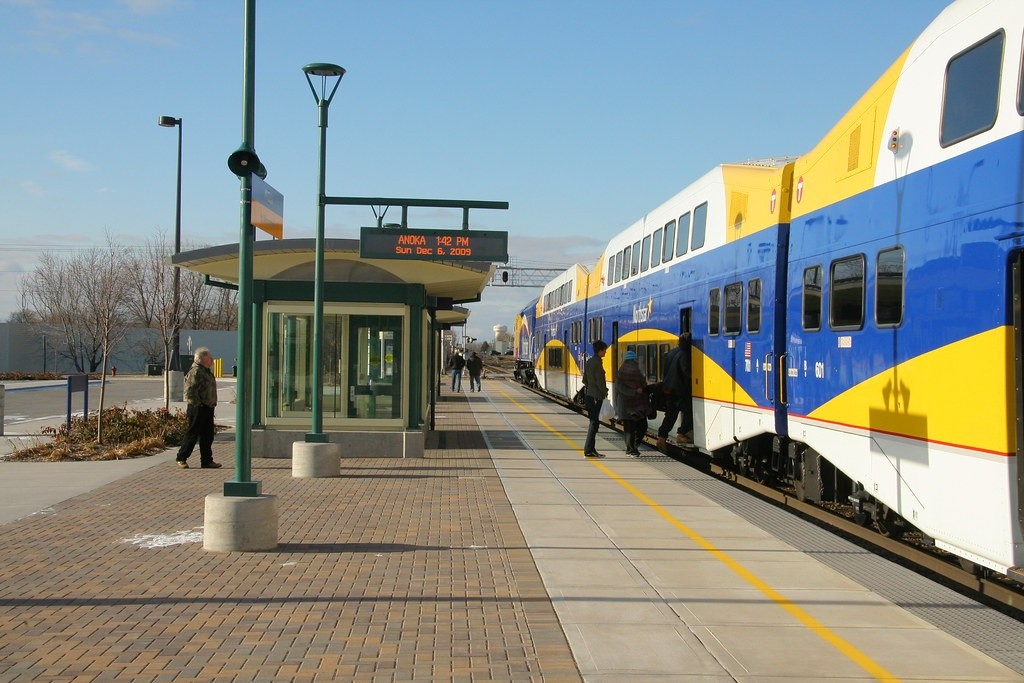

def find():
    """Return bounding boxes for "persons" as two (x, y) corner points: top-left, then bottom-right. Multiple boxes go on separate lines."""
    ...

(175, 346), (223, 471)
(467, 352), (483, 392)
(616, 350), (648, 459)
(448, 350), (465, 393)
(582, 340), (608, 459)
(656, 332), (694, 454)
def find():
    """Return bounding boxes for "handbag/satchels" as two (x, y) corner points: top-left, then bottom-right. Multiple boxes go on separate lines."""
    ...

(675, 381), (694, 413)
(647, 382), (667, 411)
(646, 390), (658, 419)
(573, 386), (587, 410)
(624, 397), (653, 421)
(599, 395), (616, 421)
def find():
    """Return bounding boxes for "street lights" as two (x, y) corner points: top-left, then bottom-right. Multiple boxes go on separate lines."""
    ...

(158, 114), (186, 372)
(290, 62), (345, 444)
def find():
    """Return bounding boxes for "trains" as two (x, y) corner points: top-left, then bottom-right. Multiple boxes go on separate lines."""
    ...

(510, 0), (1023, 628)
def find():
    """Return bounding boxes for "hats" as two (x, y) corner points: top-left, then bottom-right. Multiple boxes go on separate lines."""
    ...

(624, 351), (638, 359)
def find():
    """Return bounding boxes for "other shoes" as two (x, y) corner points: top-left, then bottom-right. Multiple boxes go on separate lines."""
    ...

(478, 386), (481, 392)
(626, 448), (640, 458)
(584, 448), (605, 458)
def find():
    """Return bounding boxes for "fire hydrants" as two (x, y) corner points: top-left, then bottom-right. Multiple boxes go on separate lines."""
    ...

(111, 365), (118, 377)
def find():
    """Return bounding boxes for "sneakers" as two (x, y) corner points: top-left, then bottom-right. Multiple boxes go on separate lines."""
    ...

(201, 460), (222, 468)
(676, 434), (691, 442)
(175, 460), (190, 468)
(656, 437), (669, 454)
